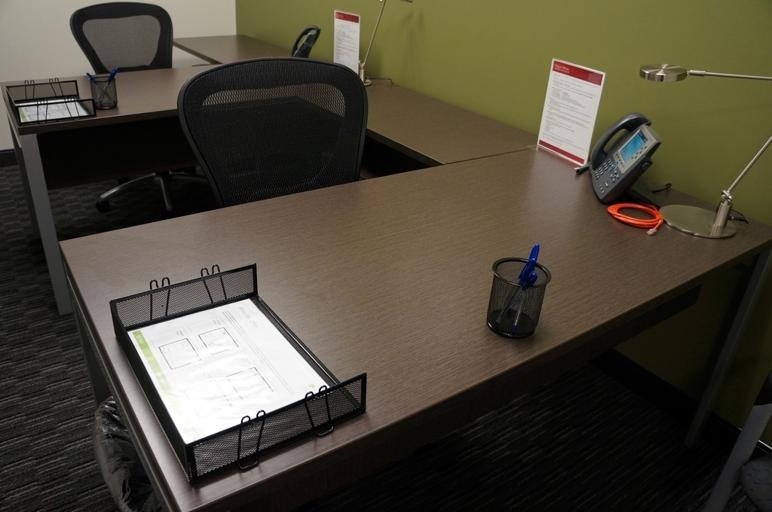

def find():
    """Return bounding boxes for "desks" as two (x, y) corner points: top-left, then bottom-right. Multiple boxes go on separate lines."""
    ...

(171, 33), (538, 182)
(0, 62), (299, 316)
(54, 147), (772, 512)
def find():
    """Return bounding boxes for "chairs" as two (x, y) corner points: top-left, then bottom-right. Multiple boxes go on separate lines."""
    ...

(69, 2), (207, 218)
(176, 56), (368, 210)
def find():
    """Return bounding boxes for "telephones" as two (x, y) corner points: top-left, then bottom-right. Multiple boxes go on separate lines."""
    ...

(589, 112), (662, 203)
(291, 26), (320, 58)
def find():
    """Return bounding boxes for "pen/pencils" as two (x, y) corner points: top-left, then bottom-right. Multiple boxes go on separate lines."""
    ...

(493, 243), (540, 334)
(86, 69), (118, 105)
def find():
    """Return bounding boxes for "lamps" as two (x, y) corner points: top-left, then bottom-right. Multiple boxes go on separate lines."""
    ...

(640, 62), (772, 241)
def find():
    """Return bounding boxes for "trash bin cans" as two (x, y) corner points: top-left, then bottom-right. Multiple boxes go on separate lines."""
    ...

(95, 396), (167, 512)
